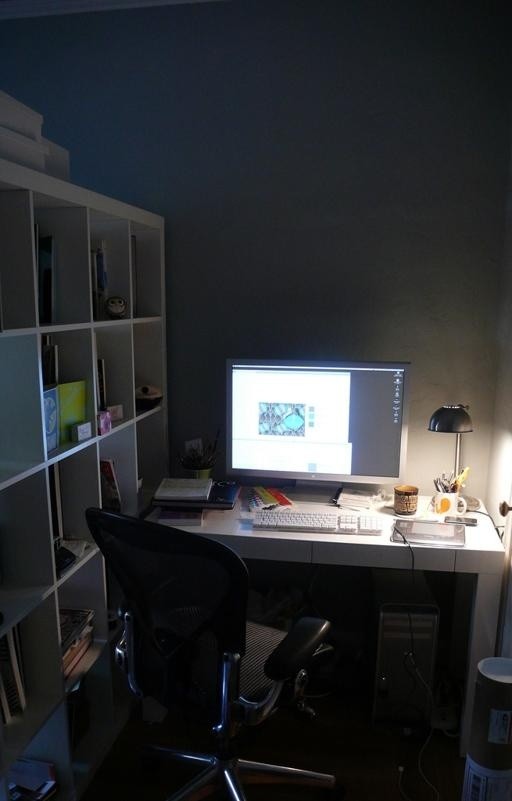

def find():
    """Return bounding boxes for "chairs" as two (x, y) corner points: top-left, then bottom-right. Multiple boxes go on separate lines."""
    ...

(84, 504), (344, 800)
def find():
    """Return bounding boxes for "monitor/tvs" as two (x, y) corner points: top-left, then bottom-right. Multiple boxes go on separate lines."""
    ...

(225, 359), (412, 505)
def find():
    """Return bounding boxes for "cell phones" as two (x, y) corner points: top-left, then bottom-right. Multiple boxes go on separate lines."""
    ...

(445, 516), (477, 526)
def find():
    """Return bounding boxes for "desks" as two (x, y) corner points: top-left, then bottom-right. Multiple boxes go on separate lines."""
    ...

(142, 486), (505, 764)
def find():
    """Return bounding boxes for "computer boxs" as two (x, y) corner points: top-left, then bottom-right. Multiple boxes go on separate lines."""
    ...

(369, 568), (440, 729)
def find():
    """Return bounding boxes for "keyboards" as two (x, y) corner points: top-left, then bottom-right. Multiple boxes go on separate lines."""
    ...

(251, 510), (382, 535)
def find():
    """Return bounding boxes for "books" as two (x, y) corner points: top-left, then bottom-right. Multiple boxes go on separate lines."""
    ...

(0, 623), (28, 726)
(36, 225), (54, 328)
(332, 487), (375, 511)
(150, 476), (243, 527)
(42, 333), (124, 580)
(58, 606), (96, 682)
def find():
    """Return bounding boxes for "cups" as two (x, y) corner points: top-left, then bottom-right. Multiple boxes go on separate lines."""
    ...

(394, 486), (417, 517)
(434, 491), (466, 522)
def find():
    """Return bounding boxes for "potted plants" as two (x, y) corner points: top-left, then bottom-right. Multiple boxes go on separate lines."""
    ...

(173, 422), (222, 479)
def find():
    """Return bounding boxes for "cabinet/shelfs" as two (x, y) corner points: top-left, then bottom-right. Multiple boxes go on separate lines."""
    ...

(0, 157), (171, 801)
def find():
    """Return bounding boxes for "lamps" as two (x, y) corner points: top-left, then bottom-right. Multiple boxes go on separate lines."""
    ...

(427, 402), (483, 512)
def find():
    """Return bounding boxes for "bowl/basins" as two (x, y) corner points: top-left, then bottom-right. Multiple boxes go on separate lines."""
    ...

(136, 394), (162, 411)
(182, 468), (213, 480)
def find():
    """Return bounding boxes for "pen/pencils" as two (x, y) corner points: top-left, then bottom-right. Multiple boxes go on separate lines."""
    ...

(434, 467), (470, 494)
(175, 428), (220, 470)
